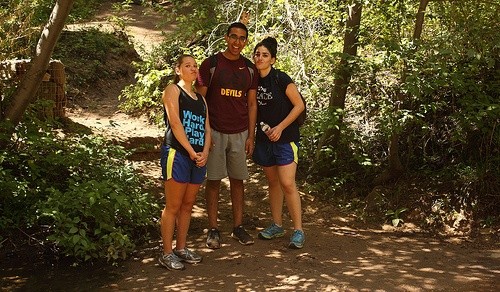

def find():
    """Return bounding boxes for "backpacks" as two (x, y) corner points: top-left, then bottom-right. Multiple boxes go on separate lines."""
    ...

(275, 68), (307, 126)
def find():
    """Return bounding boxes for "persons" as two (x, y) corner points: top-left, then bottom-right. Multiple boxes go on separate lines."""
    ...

(193, 23), (258, 250)
(157, 54), (211, 270)
(249, 37), (307, 249)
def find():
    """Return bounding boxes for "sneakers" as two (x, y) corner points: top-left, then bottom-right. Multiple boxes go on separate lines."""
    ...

(173, 247), (203, 263)
(230, 224), (254, 245)
(258, 222), (284, 240)
(205, 227), (222, 248)
(288, 229), (305, 249)
(159, 252), (185, 271)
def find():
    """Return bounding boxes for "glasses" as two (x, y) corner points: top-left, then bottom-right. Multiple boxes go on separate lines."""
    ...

(227, 35), (246, 43)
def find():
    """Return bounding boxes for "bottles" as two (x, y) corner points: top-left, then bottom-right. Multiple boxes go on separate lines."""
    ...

(260, 122), (272, 139)
